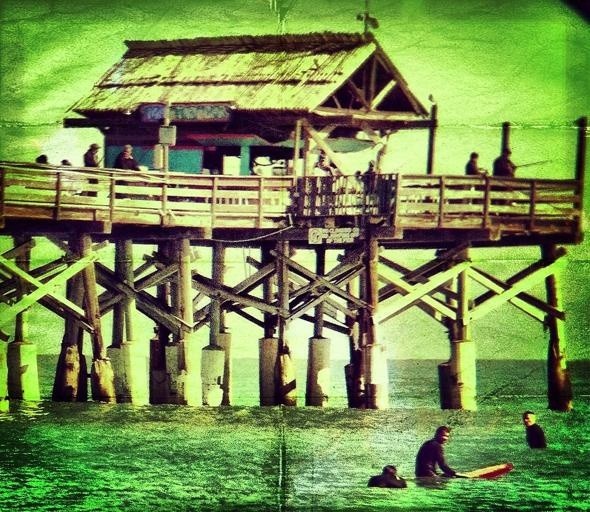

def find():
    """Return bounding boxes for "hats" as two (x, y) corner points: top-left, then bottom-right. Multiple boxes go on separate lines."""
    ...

(88, 144), (100, 151)
(503, 148), (512, 154)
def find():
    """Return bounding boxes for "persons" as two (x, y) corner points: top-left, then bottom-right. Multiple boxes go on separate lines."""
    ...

(464, 151), (489, 215)
(367, 465), (407, 488)
(522, 410), (549, 449)
(314, 153), (337, 177)
(107, 144), (141, 199)
(84, 143), (103, 198)
(365, 160), (375, 177)
(415, 427), (457, 478)
(492, 145), (518, 216)
